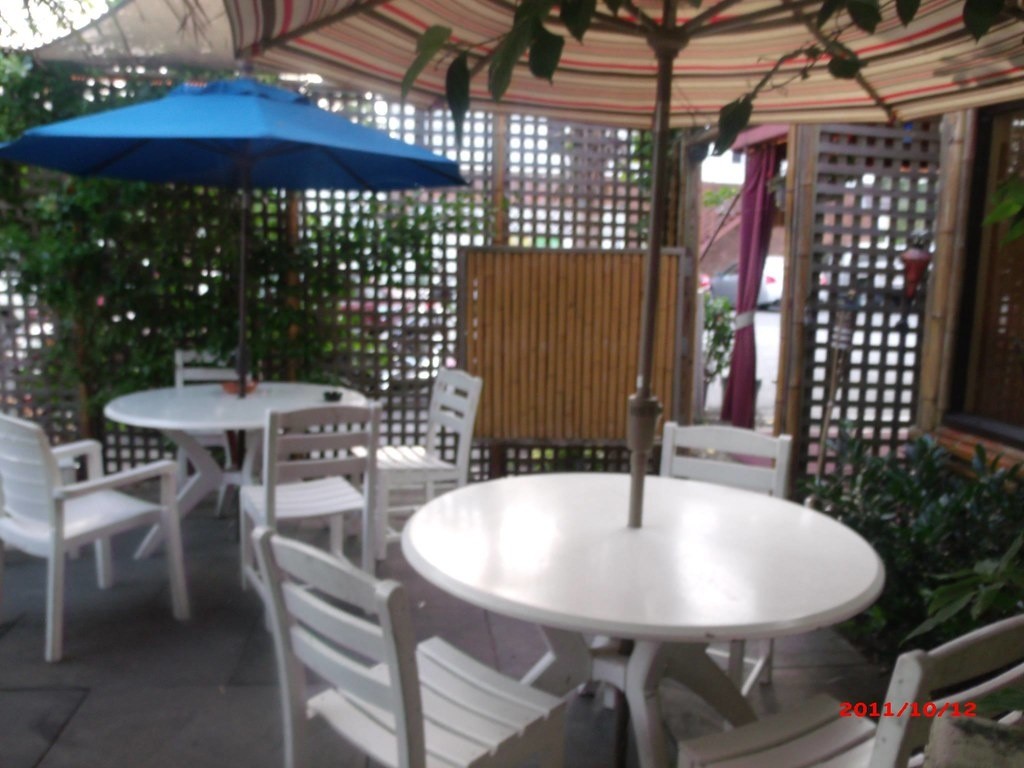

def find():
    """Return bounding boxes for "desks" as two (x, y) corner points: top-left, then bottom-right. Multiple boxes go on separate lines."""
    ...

(400, 471), (885, 768)
(104, 382), (369, 560)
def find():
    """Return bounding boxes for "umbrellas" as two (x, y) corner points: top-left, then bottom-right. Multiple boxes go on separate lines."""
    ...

(1, 57), (473, 398)
(16, 1), (1024, 529)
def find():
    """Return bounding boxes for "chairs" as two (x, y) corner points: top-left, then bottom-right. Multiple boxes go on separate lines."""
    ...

(602, 420), (792, 728)
(251, 526), (566, 768)
(172, 349), (265, 487)
(348, 364), (483, 561)
(239, 400), (382, 632)
(675, 613), (1024, 768)
(0, 413), (190, 662)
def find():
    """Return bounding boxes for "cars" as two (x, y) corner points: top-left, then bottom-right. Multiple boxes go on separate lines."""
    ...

(838, 242), (910, 293)
(333, 262), (443, 339)
(709, 253), (827, 309)
